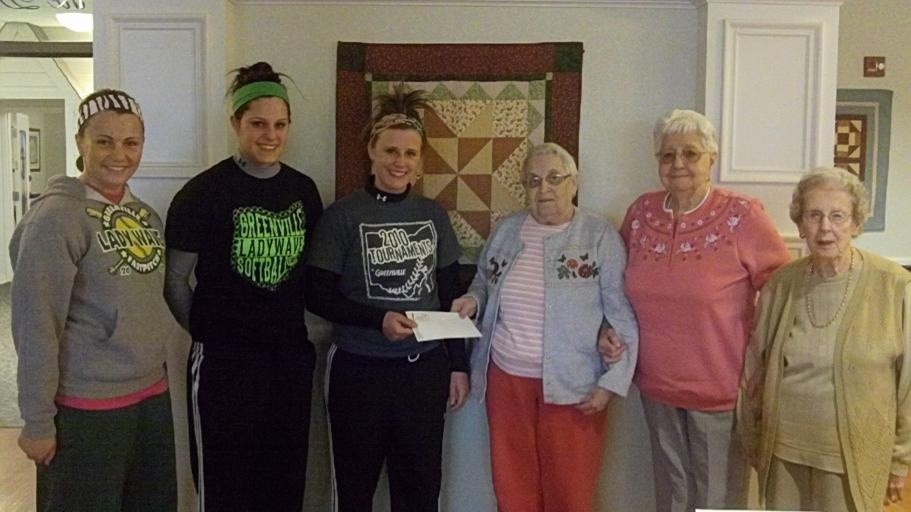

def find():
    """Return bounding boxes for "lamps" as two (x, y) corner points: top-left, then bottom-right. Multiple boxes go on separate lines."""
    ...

(54, 8), (94, 34)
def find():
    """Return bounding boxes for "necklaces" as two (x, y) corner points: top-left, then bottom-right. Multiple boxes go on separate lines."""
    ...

(805, 245), (855, 330)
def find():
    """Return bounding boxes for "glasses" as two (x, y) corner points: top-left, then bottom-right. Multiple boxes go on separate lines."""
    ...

(805, 210), (853, 223)
(523, 173), (571, 188)
(654, 147), (710, 161)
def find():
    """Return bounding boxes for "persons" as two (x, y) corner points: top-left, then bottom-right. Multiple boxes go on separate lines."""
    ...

(8, 87), (178, 511)
(729, 166), (911, 511)
(450, 141), (639, 512)
(302, 75), (470, 511)
(163, 62), (324, 511)
(619, 108), (790, 512)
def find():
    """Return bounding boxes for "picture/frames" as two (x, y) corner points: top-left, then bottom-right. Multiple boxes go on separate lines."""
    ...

(332, 42), (583, 293)
(29, 128), (41, 171)
(834, 86), (892, 233)
